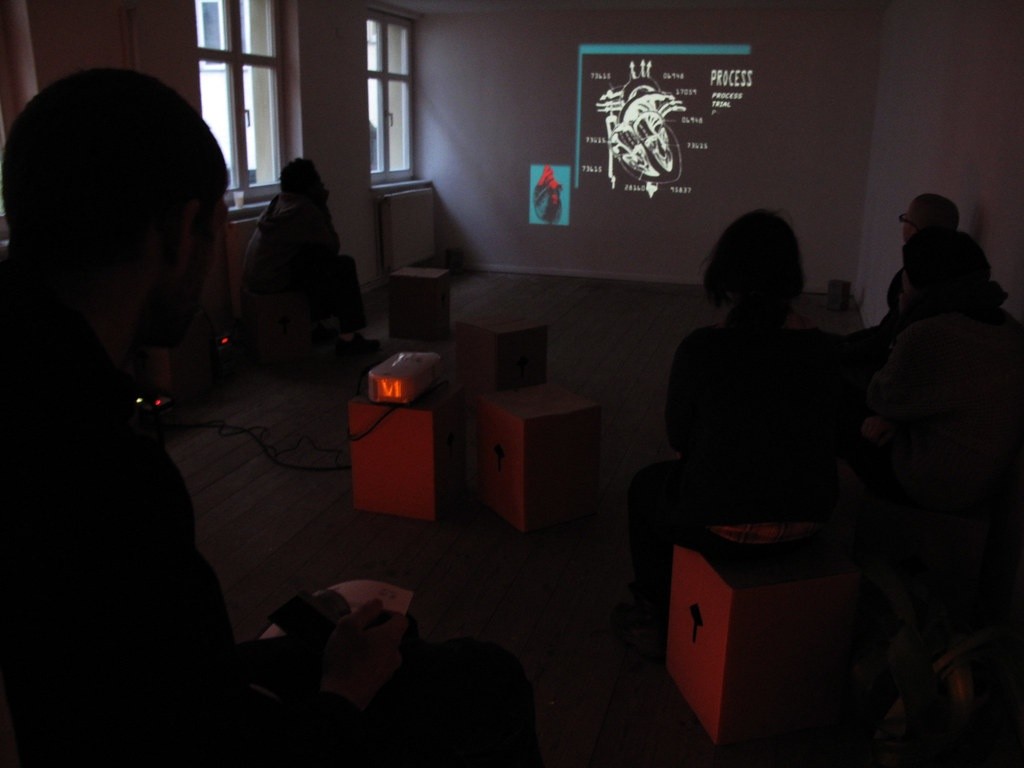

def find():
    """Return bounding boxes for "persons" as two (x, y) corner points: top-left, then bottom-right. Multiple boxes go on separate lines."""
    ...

(610, 208), (877, 662)
(242, 158), (380, 356)
(837, 193), (1024, 512)
(0, 67), (546, 768)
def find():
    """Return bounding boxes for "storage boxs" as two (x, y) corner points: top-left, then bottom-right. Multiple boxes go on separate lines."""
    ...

(390, 267), (449, 341)
(476, 383), (601, 534)
(456, 313), (548, 401)
(850, 492), (992, 579)
(244, 300), (310, 369)
(349, 390), (467, 521)
(664, 534), (862, 746)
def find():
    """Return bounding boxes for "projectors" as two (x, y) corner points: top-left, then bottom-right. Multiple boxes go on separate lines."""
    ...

(369, 350), (443, 404)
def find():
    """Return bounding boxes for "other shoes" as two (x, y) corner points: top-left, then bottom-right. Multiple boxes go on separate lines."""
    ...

(336, 331), (379, 353)
(310, 323), (338, 344)
(610, 603), (664, 655)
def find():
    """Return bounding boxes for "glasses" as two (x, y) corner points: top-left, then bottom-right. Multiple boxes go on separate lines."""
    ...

(898, 213), (919, 234)
